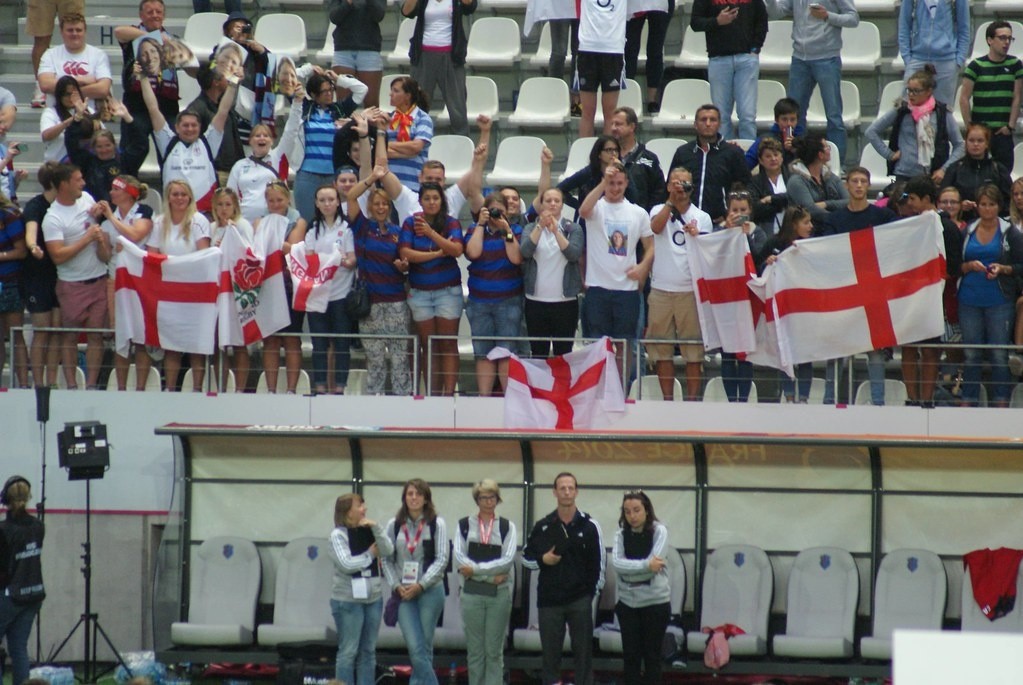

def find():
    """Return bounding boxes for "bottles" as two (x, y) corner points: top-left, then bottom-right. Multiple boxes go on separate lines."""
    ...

(448, 662), (457, 685)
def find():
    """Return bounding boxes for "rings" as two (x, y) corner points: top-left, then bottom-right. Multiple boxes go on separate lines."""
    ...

(422, 223), (424, 226)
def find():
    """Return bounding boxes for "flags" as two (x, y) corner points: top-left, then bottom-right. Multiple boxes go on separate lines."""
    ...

(114, 235), (222, 359)
(218, 213), (291, 351)
(285, 241), (342, 314)
(487, 335), (625, 428)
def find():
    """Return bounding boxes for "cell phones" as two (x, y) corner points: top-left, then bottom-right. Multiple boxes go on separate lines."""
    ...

(809, 4), (820, 9)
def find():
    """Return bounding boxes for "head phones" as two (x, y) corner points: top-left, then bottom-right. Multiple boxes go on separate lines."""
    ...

(0, 476), (31, 505)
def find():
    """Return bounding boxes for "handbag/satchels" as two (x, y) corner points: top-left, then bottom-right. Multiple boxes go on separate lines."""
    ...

(352, 278), (372, 317)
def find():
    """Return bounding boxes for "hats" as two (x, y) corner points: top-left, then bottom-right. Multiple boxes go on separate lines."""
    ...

(223, 10), (253, 34)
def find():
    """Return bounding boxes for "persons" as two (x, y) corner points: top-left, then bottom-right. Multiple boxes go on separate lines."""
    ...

(612, 490), (670, 685)
(453, 478), (516, 685)
(329, 493), (393, 685)
(274, 56), (298, 98)
(211, 42), (243, 80)
(0, 0), (1023, 407)
(523, 472), (606, 685)
(0, 475), (46, 685)
(684, 227), (761, 353)
(748, 209), (946, 380)
(163, 40), (194, 68)
(135, 37), (163, 80)
(380, 479), (450, 685)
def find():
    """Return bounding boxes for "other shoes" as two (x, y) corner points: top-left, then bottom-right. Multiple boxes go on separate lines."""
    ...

(647, 101), (661, 115)
(571, 101), (582, 116)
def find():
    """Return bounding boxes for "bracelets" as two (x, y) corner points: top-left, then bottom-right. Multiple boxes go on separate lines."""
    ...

(30, 244), (36, 250)
(666, 201), (673, 206)
(377, 129), (385, 136)
(537, 225), (543, 230)
(359, 134), (368, 138)
(3, 251), (7, 255)
(364, 179), (371, 187)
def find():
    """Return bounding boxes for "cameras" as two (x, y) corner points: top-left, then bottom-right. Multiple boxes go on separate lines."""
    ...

(487, 207), (502, 218)
(13, 143), (28, 152)
(241, 25), (251, 34)
(680, 181), (691, 192)
(735, 215), (748, 225)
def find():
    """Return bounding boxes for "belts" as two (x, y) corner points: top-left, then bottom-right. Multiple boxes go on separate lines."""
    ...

(82, 274), (106, 284)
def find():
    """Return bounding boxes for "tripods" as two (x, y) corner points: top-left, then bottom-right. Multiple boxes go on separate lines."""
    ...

(44, 479), (133, 685)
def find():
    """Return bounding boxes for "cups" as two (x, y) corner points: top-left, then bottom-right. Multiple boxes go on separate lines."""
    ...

(783, 127), (794, 149)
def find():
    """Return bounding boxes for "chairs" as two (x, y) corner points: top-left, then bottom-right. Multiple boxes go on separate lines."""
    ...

(132, 0), (1023, 407)
(168, 534), (1020, 663)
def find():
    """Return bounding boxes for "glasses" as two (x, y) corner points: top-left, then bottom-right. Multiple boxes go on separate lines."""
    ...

(478, 495), (496, 502)
(993, 35), (1014, 43)
(624, 488), (643, 496)
(214, 188), (232, 193)
(822, 145), (832, 151)
(939, 199), (962, 206)
(905, 88), (925, 95)
(421, 181), (440, 188)
(318, 87), (335, 95)
(729, 191), (750, 198)
(603, 147), (619, 154)
(266, 182), (289, 191)
(792, 205), (802, 223)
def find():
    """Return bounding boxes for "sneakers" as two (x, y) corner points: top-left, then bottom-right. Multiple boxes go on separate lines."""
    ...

(31, 91), (46, 107)
